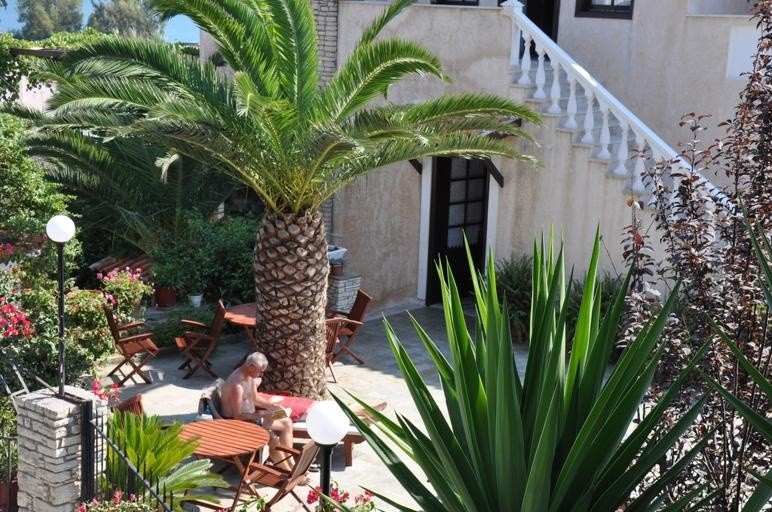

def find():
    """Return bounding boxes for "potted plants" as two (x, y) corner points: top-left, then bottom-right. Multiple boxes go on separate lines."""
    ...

(143, 208), (226, 307)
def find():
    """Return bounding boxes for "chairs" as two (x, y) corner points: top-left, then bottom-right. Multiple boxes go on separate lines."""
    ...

(200, 377), (366, 468)
(320, 318), (341, 381)
(321, 288), (371, 364)
(173, 298), (227, 380)
(108, 391), (178, 437)
(244, 437), (319, 511)
(102, 302), (159, 387)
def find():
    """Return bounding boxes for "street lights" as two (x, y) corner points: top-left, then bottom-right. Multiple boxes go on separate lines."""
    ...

(46, 214), (75, 399)
(306, 400), (350, 511)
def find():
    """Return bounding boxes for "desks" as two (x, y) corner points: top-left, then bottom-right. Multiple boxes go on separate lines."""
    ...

(223, 301), (322, 375)
(175, 419), (270, 512)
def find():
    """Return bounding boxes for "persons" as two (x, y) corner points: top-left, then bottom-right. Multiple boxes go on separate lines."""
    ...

(221, 352), (312, 487)
